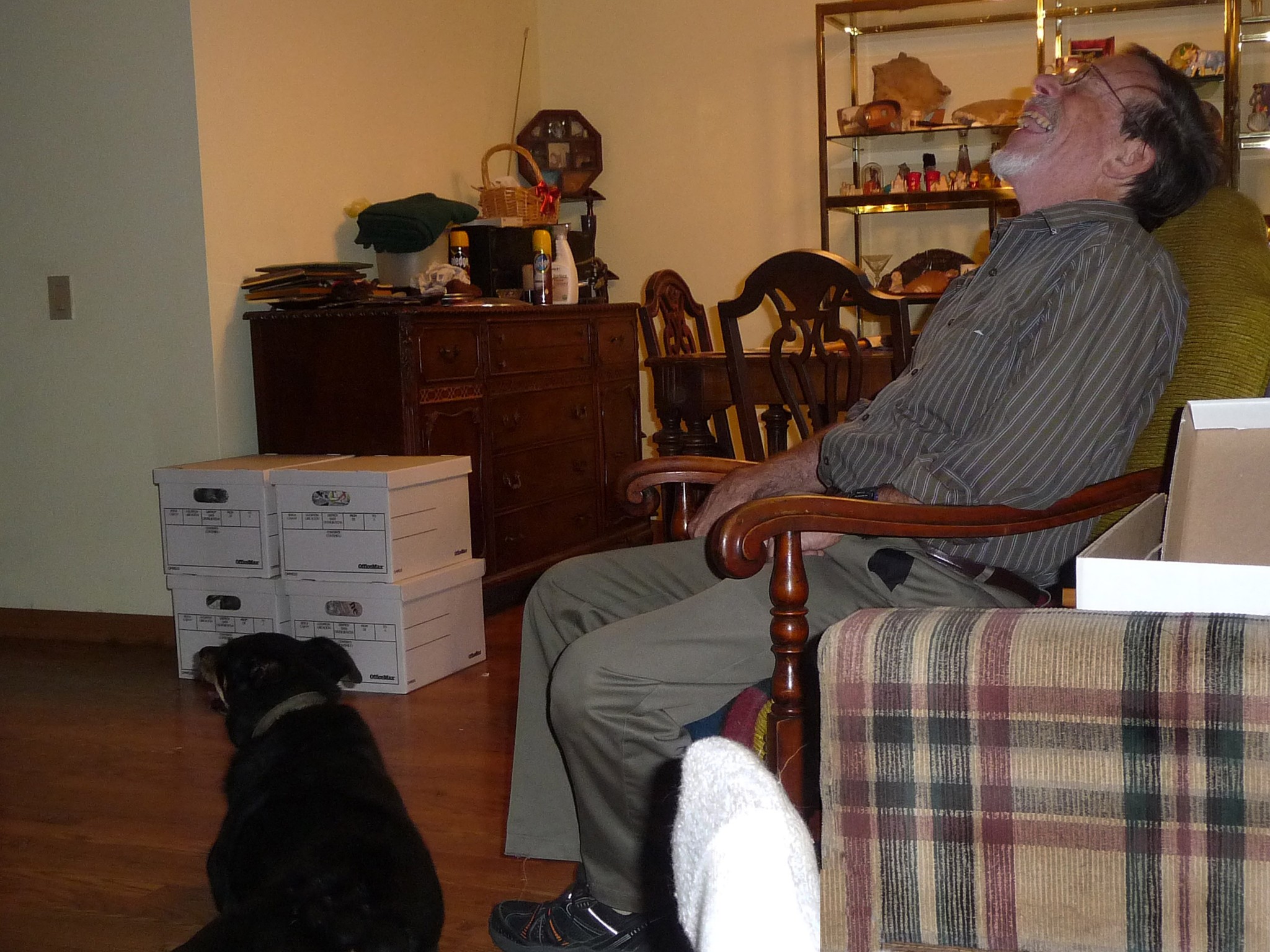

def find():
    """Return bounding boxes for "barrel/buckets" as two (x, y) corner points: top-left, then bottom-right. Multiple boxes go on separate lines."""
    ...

(376, 221), (452, 285)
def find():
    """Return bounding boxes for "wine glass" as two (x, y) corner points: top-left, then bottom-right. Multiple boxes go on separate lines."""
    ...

(860, 254), (893, 288)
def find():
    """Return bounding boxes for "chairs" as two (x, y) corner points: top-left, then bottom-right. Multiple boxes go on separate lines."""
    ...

(718, 248), (913, 462)
(638, 268), (736, 460)
(615, 176), (1268, 824)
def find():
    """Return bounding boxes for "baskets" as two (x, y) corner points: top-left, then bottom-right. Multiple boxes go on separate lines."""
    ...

(470, 143), (559, 228)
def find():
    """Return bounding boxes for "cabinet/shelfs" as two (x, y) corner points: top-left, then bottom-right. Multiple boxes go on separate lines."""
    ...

(245, 302), (653, 593)
(817, 0), (1270, 339)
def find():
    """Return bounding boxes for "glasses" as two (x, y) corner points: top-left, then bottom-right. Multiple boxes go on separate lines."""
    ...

(1059, 59), (1153, 148)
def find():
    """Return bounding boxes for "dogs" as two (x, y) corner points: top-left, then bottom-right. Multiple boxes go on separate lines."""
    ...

(200, 632), (444, 952)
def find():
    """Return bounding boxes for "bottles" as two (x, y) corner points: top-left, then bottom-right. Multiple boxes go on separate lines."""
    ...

(532, 230), (553, 307)
(955, 144), (972, 176)
(450, 231), (471, 293)
(989, 142), (1002, 188)
(551, 231), (579, 305)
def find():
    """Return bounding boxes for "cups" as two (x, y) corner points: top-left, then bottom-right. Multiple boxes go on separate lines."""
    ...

(960, 263), (978, 275)
(906, 170), (996, 192)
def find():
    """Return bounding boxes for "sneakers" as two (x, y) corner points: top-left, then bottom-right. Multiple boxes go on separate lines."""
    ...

(486, 878), (670, 952)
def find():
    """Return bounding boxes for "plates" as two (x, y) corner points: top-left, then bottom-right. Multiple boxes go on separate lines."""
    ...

(368, 284), (475, 304)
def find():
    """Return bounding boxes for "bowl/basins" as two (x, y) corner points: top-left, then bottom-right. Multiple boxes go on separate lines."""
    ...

(496, 288), (523, 300)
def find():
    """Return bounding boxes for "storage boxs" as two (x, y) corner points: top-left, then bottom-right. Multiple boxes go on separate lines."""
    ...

(268, 454), (472, 584)
(1075, 394), (1268, 618)
(164, 566), (291, 680)
(281, 557), (485, 694)
(153, 453), (354, 577)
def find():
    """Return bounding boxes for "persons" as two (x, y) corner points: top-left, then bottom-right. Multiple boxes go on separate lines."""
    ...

(948, 170), (966, 191)
(487, 41), (1217, 952)
